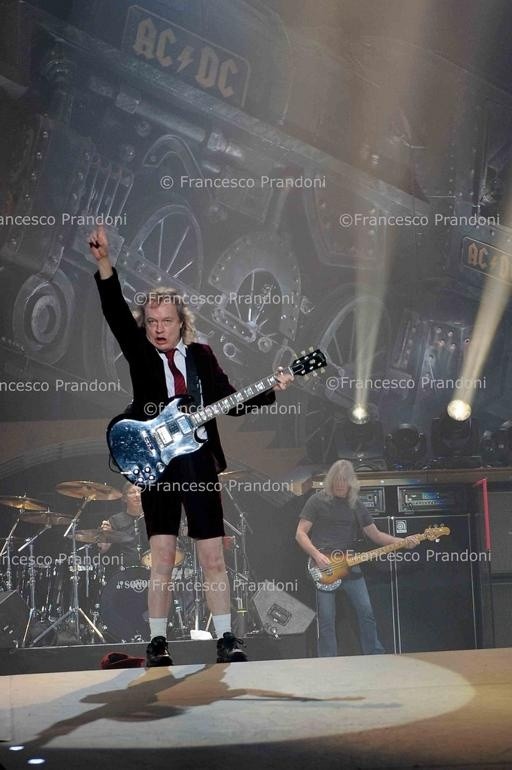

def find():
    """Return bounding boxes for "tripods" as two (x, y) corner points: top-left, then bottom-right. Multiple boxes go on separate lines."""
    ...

(0, 494), (107, 648)
(166, 476), (254, 639)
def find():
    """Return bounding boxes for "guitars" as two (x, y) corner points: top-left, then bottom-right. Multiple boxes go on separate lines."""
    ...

(307, 524), (450, 592)
(110, 349), (327, 487)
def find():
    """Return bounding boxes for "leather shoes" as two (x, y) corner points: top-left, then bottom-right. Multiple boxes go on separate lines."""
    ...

(215, 632), (249, 663)
(145, 636), (174, 667)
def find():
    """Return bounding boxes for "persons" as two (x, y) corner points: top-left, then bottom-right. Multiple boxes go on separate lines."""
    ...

(295, 459), (420, 657)
(97, 481), (150, 553)
(87, 223), (295, 667)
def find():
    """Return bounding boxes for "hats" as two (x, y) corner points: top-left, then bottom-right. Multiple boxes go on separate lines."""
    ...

(99, 652), (145, 670)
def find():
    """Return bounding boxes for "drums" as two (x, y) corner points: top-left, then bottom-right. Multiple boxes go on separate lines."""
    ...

(133, 512), (187, 570)
(99, 564), (180, 643)
(171, 568), (196, 613)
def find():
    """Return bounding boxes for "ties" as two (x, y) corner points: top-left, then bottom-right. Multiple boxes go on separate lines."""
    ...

(164, 348), (188, 395)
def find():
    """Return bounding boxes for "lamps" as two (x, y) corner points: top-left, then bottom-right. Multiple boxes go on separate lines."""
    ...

(343, 400), (512, 467)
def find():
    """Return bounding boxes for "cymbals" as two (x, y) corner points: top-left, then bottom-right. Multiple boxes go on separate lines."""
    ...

(0, 496), (54, 510)
(66, 530), (134, 543)
(55, 481), (123, 501)
(17, 512), (80, 526)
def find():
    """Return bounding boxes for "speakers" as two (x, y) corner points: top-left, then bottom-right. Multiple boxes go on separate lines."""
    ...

(249, 582), (315, 635)
(479, 491), (511, 579)
(313, 515), (396, 657)
(481, 582), (512, 650)
(392, 513), (478, 654)
